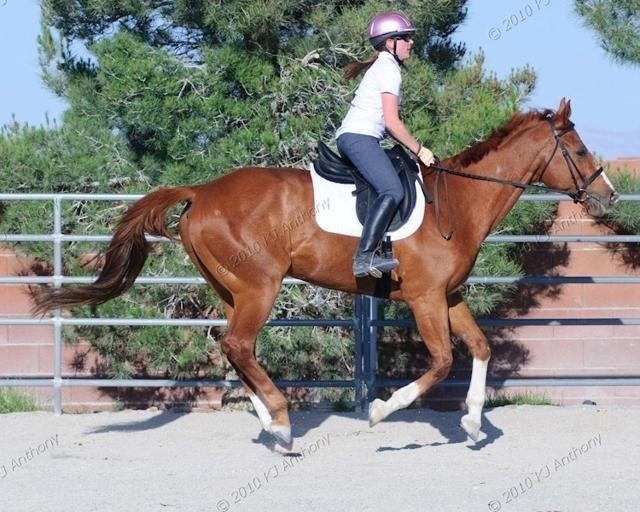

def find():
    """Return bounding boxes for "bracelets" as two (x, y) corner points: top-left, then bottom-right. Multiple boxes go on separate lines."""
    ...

(416, 142), (421, 155)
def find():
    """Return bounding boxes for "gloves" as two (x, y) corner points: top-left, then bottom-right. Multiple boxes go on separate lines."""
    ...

(417, 145), (436, 168)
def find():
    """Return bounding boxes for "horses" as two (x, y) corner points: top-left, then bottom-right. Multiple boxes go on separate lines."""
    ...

(25, 97), (622, 445)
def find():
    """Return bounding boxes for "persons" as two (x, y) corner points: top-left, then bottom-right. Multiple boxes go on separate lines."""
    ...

(334, 13), (436, 279)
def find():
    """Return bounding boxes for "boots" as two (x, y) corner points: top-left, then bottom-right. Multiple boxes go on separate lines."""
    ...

(352, 193), (400, 277)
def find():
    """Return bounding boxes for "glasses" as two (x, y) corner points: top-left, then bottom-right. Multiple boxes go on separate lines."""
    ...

(391, 34), (411, 43)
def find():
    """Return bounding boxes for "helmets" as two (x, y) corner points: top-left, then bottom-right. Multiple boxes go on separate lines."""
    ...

(367, 11), (417, 49)
